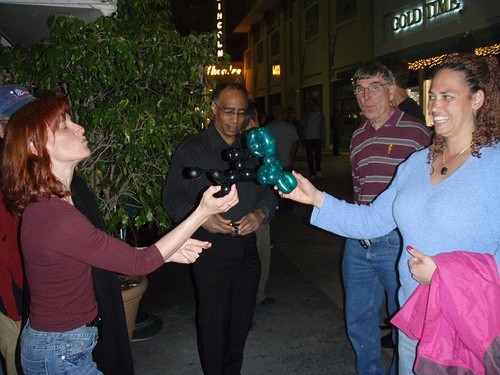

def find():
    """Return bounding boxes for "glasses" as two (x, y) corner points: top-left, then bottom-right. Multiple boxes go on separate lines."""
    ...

(211, 98), (250, 118)
(354, 81), (392, 95)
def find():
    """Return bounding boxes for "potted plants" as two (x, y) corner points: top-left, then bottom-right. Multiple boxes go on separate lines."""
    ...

(0, 0), (244, 350)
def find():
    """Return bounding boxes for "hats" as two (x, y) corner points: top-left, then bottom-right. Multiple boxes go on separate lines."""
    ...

(0, 83), (37, 118)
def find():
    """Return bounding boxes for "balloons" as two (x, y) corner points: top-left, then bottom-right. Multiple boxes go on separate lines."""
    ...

(246, 126), (297, 194)
(188, 146), (262, 197)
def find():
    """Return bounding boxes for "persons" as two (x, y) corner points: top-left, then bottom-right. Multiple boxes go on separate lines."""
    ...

(275, 53), (500, 375)
(240, 98), (344, 306)
(379, 57), (426, 125)
(0, 81), (134, 375)
(341, 59), (435, 375)
(0, 91), (239, 375)
(161, 81), (281, 375)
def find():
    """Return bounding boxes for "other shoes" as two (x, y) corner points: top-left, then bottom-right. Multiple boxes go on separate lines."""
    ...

(249, 321), (257, 332)
(262, 296), (276, 307)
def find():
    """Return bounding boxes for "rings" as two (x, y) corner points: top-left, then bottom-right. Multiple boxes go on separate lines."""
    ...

(226, 202), (230, 206)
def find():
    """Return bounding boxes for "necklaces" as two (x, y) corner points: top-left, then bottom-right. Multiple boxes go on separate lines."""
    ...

(440, 144), (472, 174)
